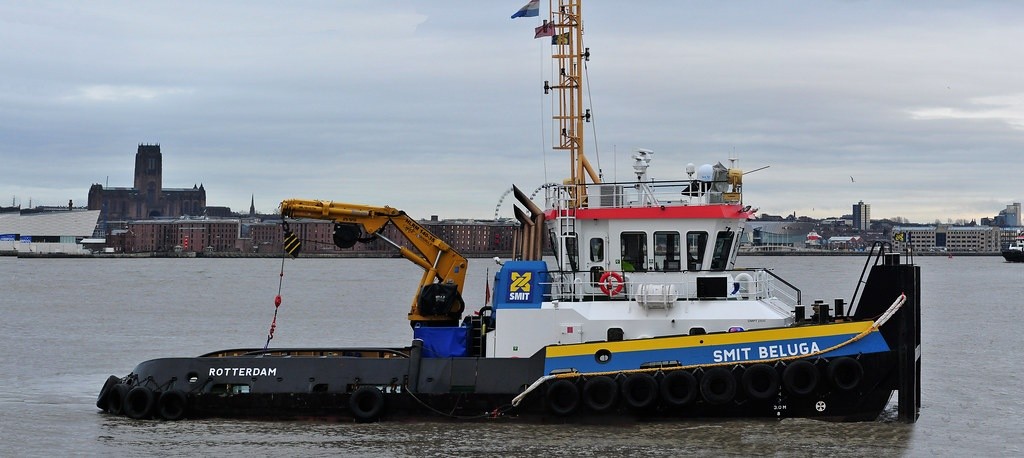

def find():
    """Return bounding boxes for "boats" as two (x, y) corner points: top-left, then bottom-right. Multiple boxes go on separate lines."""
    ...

(1002, 231), (1024, 262)
(95, 0), (922, 423)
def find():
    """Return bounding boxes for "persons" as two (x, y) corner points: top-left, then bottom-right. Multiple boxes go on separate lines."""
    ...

(731, 283), (740, 294)
(622, 256), (640, 272)
(595, 239), (601, 259)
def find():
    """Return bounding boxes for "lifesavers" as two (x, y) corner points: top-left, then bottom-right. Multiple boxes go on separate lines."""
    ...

(542, 356), (865, 416)
(94, 373), (387, 425)
(599, 271), (624, 296)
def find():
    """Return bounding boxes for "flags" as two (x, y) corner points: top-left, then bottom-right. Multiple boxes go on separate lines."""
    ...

(486, 283), (490, 302)
(552, 32), (569, 45)
(535, 21), (555, 37)
(511, 0), (539, 19)
